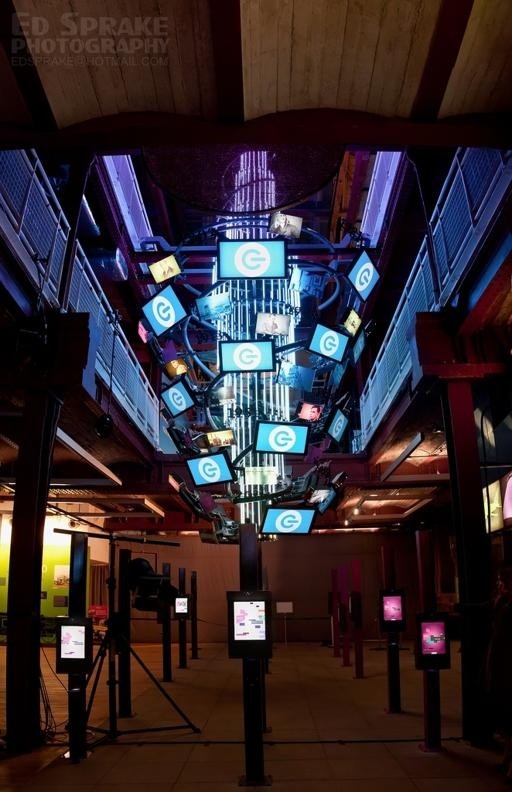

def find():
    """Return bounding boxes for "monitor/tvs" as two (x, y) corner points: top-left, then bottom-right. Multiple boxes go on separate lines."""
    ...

(480, 470), (512, 534)
(216, 238), (288, 280)
(168, 471), (185, 492)
(158, 377), (199, 419)
(309, 487), (337, 515)
(325, 406), (350, 444)
(140, 282), (192, 338)
(218, 340), (277, 373)
(138, 318), (155, 344)
(256, 312), (292, 336)
(148, 254), (183, 284)
(304, 322), (351, 363)
(345, 248), (383, 303)
(275, 359), (316, 393)
(165, 356), (191, 379)
(206, 428), (237, 448)
(243, 466), (278, 486)
(339, 306), (364, 338)
(253, 419), (312, 456)
(195, 292), (233, 321)
(185, 449), (239, 487)
(259, 506), (318, 536)
(267, 211), (303, 239)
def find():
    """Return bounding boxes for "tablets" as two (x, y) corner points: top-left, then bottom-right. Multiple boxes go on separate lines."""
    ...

(55, 592), (448, 660)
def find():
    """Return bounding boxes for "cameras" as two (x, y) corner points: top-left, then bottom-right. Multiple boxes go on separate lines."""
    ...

(295, 399), (325, 422)
